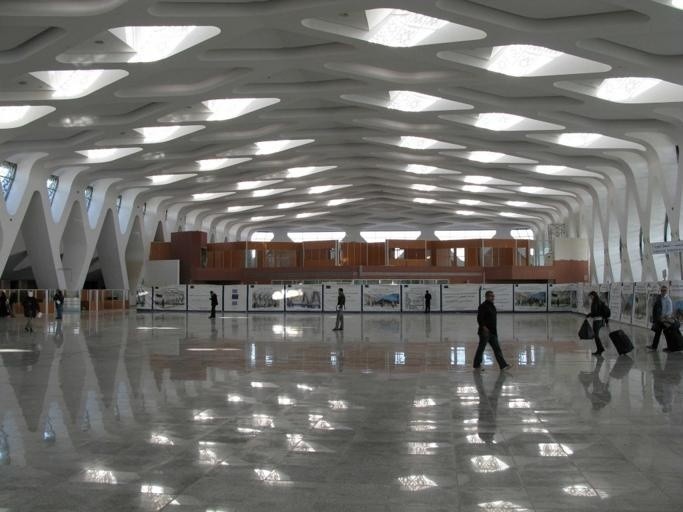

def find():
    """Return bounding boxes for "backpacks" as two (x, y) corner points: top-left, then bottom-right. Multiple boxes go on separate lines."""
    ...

(598, 300), (611, 319)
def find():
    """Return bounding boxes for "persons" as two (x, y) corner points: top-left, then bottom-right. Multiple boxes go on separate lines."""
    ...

(332, 289), (345, 330)
(208, 291), (218, 318)
(473, 290), (513, 371)
(425, 290), (431, 312)
(585, 290), (605, 355)
(0, 289), (64, 332)
(646, 285), (673, 351)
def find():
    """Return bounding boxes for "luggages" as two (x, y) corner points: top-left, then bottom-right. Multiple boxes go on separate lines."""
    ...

(608, 330), (633, 355)
(664, 321), (682, 352)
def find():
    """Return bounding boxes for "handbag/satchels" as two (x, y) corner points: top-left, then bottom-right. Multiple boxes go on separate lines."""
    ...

(577, 319), (595, 339)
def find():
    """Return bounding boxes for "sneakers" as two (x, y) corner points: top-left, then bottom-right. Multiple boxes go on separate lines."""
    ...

(474, 366), (484, 371)
(501, 362), (513, 371)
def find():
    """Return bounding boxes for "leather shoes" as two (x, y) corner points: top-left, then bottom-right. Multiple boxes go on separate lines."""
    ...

(592, 348), (603, 355)
